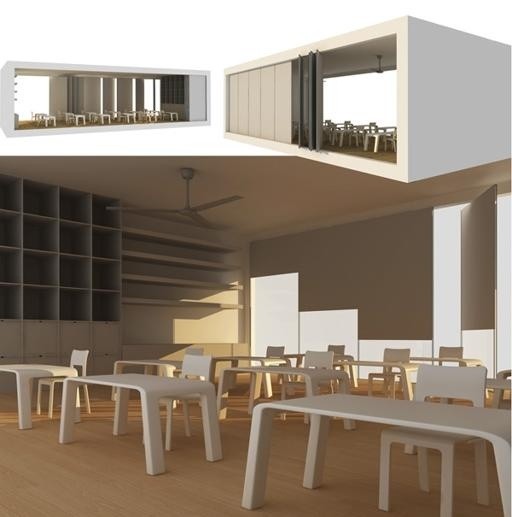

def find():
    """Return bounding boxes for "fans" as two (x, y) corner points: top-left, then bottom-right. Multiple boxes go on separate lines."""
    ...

(376, 55), (384, 73)
(107, 168), (244, 228)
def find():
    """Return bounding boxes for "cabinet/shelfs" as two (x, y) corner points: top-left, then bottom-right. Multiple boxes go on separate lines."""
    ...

(121, 227), (244, 309)
(0, 172), (121, 400)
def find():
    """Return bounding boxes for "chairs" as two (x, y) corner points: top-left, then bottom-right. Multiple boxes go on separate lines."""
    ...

(31, 109), (178, 127)
(379, 363), (489, 517)
(323, 120), (397, 153)
(37, 349), (91, 419)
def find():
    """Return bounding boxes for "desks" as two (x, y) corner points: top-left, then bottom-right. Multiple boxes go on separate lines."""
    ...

(0, 364), (81, 429)
(59, 373), (223, 475)
(241, 393), (511, 517)
